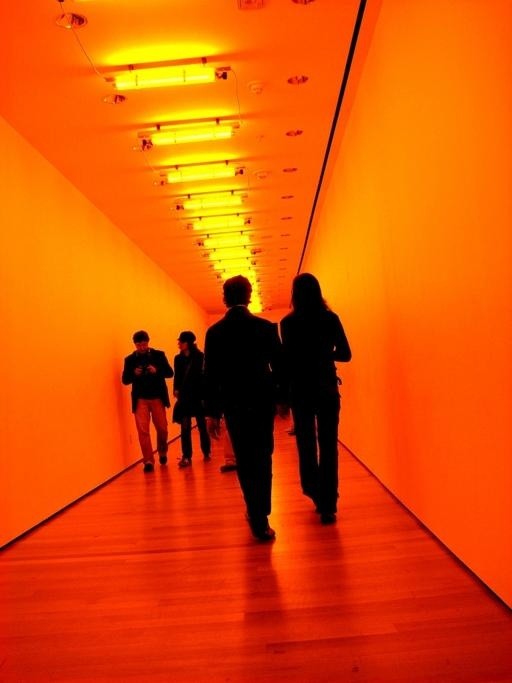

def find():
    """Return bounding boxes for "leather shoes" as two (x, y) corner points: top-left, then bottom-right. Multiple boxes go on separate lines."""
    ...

(160, 456), (167, 465)
(257, 529), (275, 544)
(144, 463), (154, 472)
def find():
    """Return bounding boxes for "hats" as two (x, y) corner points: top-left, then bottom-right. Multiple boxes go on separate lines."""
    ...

(177, 332), (196, 341)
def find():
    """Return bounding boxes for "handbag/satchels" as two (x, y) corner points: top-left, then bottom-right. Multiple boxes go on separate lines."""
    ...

(174, 400), (182, 424)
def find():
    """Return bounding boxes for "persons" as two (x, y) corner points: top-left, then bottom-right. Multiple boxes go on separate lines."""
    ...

(120, 330), (175, 473)
(201, 274), (290, 544)
(218, 430), (237, 472)
(278, 272), (352, 523)
(170, 330), (211, 467)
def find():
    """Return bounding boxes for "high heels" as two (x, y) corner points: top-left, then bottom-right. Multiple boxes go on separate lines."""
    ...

(315, 505), (336, 524)
(177, 457), (192, 467)
